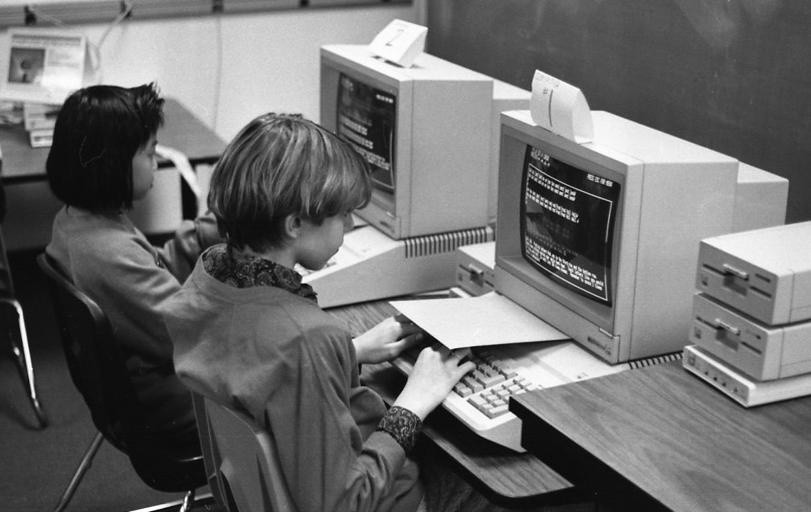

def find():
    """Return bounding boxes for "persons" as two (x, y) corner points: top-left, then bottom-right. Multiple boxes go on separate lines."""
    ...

(151, 112), (503, 510)
(42, 72), (224, 463)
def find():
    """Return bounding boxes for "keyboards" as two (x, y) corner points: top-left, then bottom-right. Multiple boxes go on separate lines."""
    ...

(294, 225), (494, 310)
(388, 312), (683, 454)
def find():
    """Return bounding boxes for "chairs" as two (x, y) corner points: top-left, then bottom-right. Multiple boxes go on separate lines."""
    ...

(33, 256), (221, 512)
(131, 383), (297, 511)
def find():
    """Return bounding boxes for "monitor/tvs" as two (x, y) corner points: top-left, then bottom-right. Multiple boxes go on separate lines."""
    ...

(493, 110), (789, 365)
(319, 45), (532, 241)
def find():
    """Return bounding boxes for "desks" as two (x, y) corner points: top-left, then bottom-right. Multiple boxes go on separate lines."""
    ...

(0, 97), (232, 364)
(326, 297), (608, 509)
(507, 361), (809, 510)
